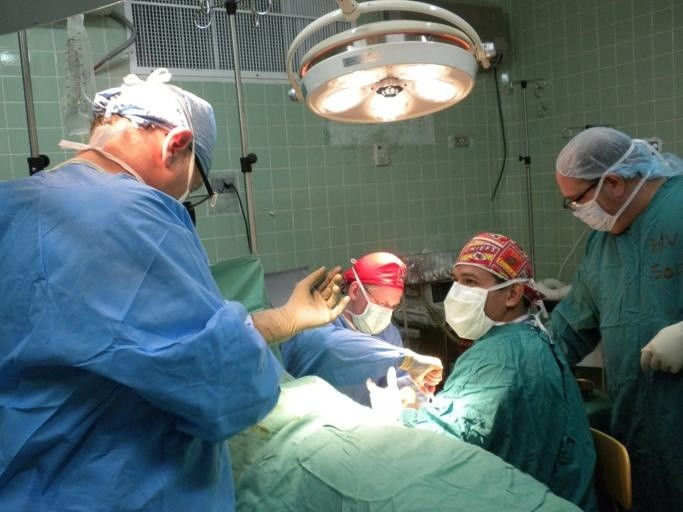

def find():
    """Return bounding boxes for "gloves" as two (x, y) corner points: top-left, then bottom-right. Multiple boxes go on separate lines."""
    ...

(252, 264), (350, 343)
(640, 320), (682, 375)
(400, 350), (443, 396)
(366, 367), (406, 423)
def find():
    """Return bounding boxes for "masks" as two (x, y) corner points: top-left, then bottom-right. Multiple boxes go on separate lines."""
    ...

(444, 278), (527, 341)
(571, 137), (626, 234)
(344, 265), (394, 334)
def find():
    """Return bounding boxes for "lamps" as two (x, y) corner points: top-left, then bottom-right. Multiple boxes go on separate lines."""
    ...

(284, 0), (495, 124)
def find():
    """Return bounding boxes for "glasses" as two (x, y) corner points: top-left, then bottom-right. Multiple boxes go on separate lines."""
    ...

(363, 285), (401, 310)
(189, 144), (213, 207)
(563, 177), (599, 209)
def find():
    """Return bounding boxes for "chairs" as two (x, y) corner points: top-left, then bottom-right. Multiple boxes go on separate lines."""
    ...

(588, 426), (633, 511)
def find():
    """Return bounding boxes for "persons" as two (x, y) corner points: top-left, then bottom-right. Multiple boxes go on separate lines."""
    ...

(0, 65), (357, 510)
(363, 231), (603, 511)
(537, 127), (683, 511)
(276, 250), (443, 411)
